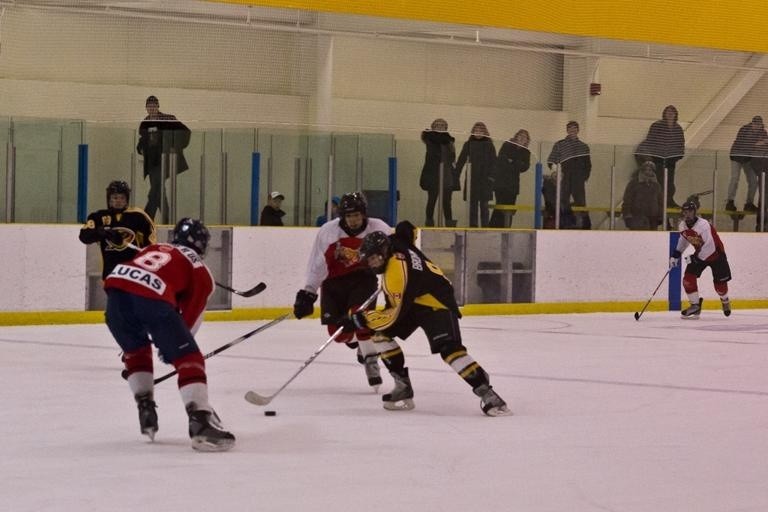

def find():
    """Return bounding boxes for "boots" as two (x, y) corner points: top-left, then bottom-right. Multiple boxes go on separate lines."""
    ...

(134, 391), (159, 433)
(365, 354), (382, 386)
(720, 298), (731, 316)
(473, 382), (505, 412)
(681, 298), (703, 316)
(381, 365), (414, 401)
(186, 401), (236, 440)
(346, 340), (365, 364)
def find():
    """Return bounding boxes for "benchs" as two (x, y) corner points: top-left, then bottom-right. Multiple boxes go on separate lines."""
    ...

(480, 203), (764, 230)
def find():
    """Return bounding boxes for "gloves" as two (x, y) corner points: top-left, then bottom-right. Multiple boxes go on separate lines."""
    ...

(669, 250), (681, 268)
(293, 289), (318, 319)
(684, 255), (697, 264)
(103, 229), (124, 245)
(336, 311), (367, 333)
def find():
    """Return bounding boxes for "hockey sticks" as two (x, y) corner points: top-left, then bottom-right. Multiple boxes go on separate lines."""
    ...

(245, 286), (379, 406)
(121, 312), (293, 385)
(127, 243), (266, 297)
(634, 263), (675, 320)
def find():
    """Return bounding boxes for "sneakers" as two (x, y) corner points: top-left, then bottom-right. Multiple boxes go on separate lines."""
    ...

(726, 201), (737, 211)
(744, 203), (759, 212)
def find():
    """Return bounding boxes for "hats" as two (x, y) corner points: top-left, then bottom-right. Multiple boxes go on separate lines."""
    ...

(145, 96), (159, 109)
(268, 192), (284, 201)
(642, 160), (657, 171)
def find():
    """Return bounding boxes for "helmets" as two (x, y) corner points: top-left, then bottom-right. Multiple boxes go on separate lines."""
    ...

(172, 217), (210, 254)
(360, 231), (392, 260)
(680, 202), (697, 223)
(339, 191), (368, 236)
(106, 181), (131, 211)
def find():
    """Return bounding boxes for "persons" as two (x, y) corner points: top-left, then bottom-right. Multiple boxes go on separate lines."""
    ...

(292, 191), (393, 394)
(77, 179), (158, 380)
(258, 189), (287, 227)
(635, 104), (685, 208)
(666, 199), (734, 320)
(133, 93), (192, 223)
(723, 115), (766, 212)
(102, 216), (235, 454)
(315, 195), (341, 227)
(418, 116), (594, 231)
(336, 220), (514, 418)
(621, 160), (666, 230)
